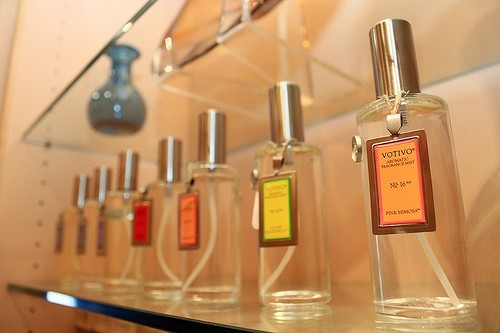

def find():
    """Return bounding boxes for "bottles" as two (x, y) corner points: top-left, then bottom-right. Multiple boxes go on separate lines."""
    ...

(104, 151), (139, 300)
(75, 166), (111, 295)
(354, 15), (477, 327)
(134, 138), (188, 305)
(87, 44), (145, 137)
(182, 109), (240, 311)
(251, 80), (333, 309)
(52, 175), (89, 292)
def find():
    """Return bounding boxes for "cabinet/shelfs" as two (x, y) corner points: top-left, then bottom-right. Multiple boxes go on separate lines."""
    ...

(10, 0), (500, 333)
